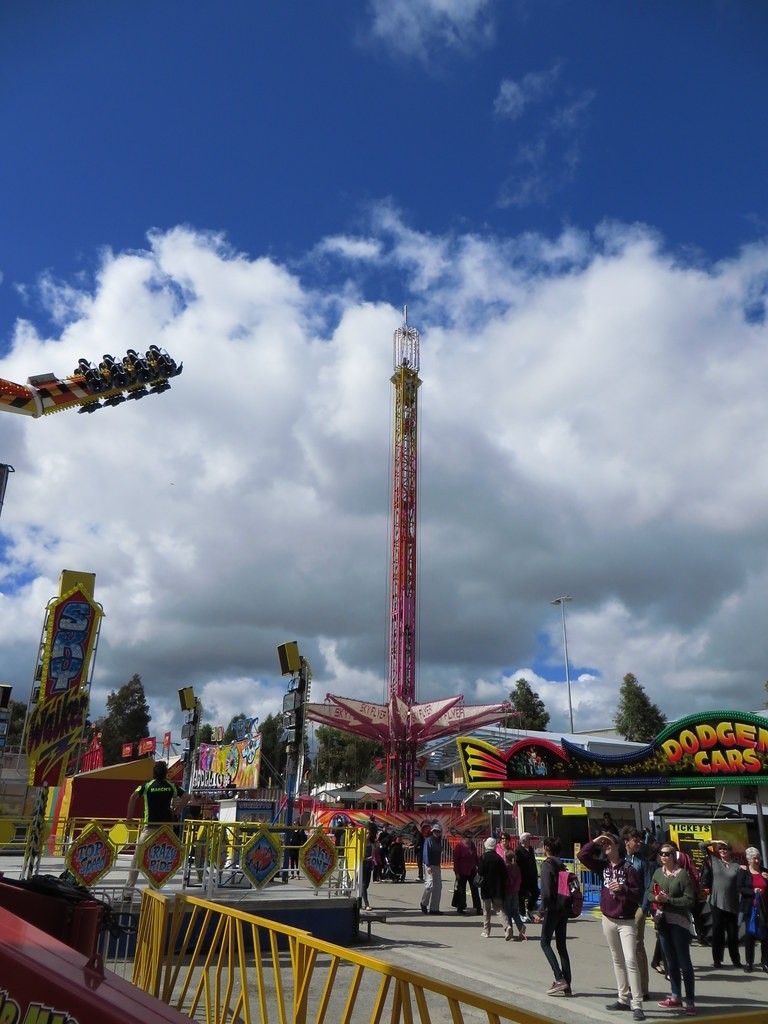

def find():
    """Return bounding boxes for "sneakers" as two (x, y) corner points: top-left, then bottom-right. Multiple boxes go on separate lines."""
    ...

(686, 1005), (699, 1015)
(547, 979), (572, 996)
(658, 998), (683, 1008)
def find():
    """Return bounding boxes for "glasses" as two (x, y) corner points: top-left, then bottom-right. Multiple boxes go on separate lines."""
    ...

(659, 851), (671, 857)
(719, 847), (726, 850)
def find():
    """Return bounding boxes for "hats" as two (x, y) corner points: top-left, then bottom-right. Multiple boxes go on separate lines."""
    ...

(602, 812), (612, 822)
(292, 817), (300, 824)
(430, 824), (443, 831)
(484, 838), (497, 849)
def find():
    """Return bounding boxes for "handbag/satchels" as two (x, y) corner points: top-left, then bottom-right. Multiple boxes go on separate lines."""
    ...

(699, 849), (712, 888)
(652, 882), (661, 910)
(473, 853), (488, 887)
(749, 891), (765, 939)
(451, 879), (468, 908)
(654, 909), (665, 930)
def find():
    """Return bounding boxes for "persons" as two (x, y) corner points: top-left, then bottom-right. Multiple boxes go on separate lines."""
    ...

(576, 832), (645, 1022)
(534, 833), (572, 998)
(451, 829), (484, 916)
(110, 761), (192, 904)
(406, 823), (424, 883)
(474, 831), (539, 943)
(590, 812), (768, 1002)
(332, 816), (406, 911)
(288, 818), (307, 880)
(420, 824), (444, 916)
(647, 844), (697, 1016)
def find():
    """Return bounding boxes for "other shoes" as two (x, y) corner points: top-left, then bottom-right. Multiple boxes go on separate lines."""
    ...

(518, 926), (527, 939)
(606, 1002), (631, 1011)
(480, 932), (489, 937)
(111, 893), (131, 904)
(429, 910), (444, 915)
(733, 962), (742, 968)
(364, 906), (373, 911)
(457, 908), (466, 914)
(633, 1009), (645, 1020)
(476, 907), (483, 915)
(504, 926), (512, 941)
(744, 962), (753, 972)
(420, 902), (427, 915)
(762, 964), (768, 973)
(714, 961), (721, 968)
(520, 910), (539, 923)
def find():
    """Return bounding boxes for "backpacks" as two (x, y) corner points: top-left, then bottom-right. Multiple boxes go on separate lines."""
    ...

(545, 858), (582, 918)
